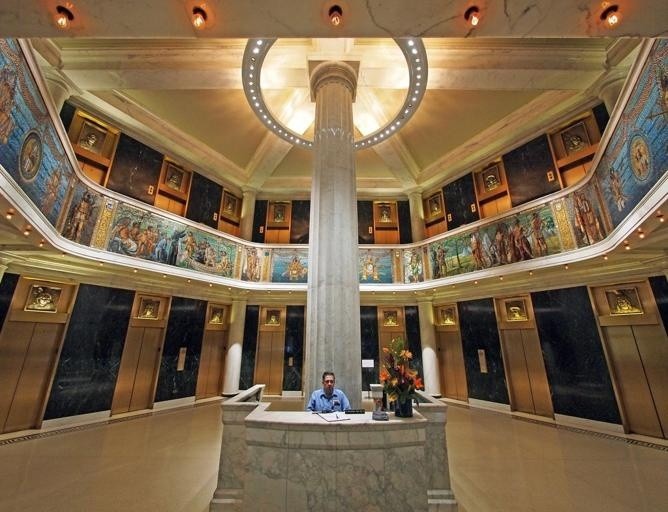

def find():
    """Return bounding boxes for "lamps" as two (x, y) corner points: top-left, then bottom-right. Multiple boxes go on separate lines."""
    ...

(599, 2), (623, 31)
(192, 4), (208, 31)
(327, 5), (344, 27)
(463, 6), (481, 27)
(55, 3), (75, 30)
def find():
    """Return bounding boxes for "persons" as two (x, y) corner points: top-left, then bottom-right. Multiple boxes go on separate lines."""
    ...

(68, 187), (98, 244)
(435, 246), (450, 277)
(166, 173), (181, 190)
(306, 371), (351, 412)
(211, 308), (224, 324)
(610, 294), (643, 315)
(466, 212), (552, 268)
(438, 309), (454, 325)
(377, 207), (391, 222)
(42, 161), (63, 219)
(360, 256), (382, 281)
(26, 287), (57, 311)
(282, 254), (308, 282)
(81, 132), (99, 151)
(565, 133), (653, 247)
(245, 248), (261, 282)
(382, 311), (397, 327)
(267, 312), (280, 325)
(111, 212), (232, 275)
(408, 247), (422, 284)
(507, 305), (528, 320)
(141, 303), (158, 318)
(0, 64), (17, 149)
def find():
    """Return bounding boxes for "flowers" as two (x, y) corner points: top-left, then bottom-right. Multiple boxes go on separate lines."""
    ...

(377, 333), (424, 409)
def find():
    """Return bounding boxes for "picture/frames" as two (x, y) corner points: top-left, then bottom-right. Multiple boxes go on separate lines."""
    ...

(481, 166), (502, 191)
(603, 286), (644, 316)
(222, 193), (238, 218)
(164, 164), (184, 192)
(383, 309), (400, 327)
(265, 310), (281, 326)
(427, 194), (443, 217)
(209, 306), (224, 325)
(439, 307), (455, 326)
(137, 297), (161, 319)
(504, 299), (529, 321)
(76, 120), (108, 155)
(24, 284), (62, 313)
(378, 205), (392, 222)
(558, 122), (592, 158)
(273, 205), (286, 222)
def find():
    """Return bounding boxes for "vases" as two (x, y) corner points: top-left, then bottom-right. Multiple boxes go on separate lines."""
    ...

(393, 389), (412, 417)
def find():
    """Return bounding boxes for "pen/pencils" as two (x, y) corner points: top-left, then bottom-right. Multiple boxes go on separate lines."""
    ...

(336, 414), (338, 419)
